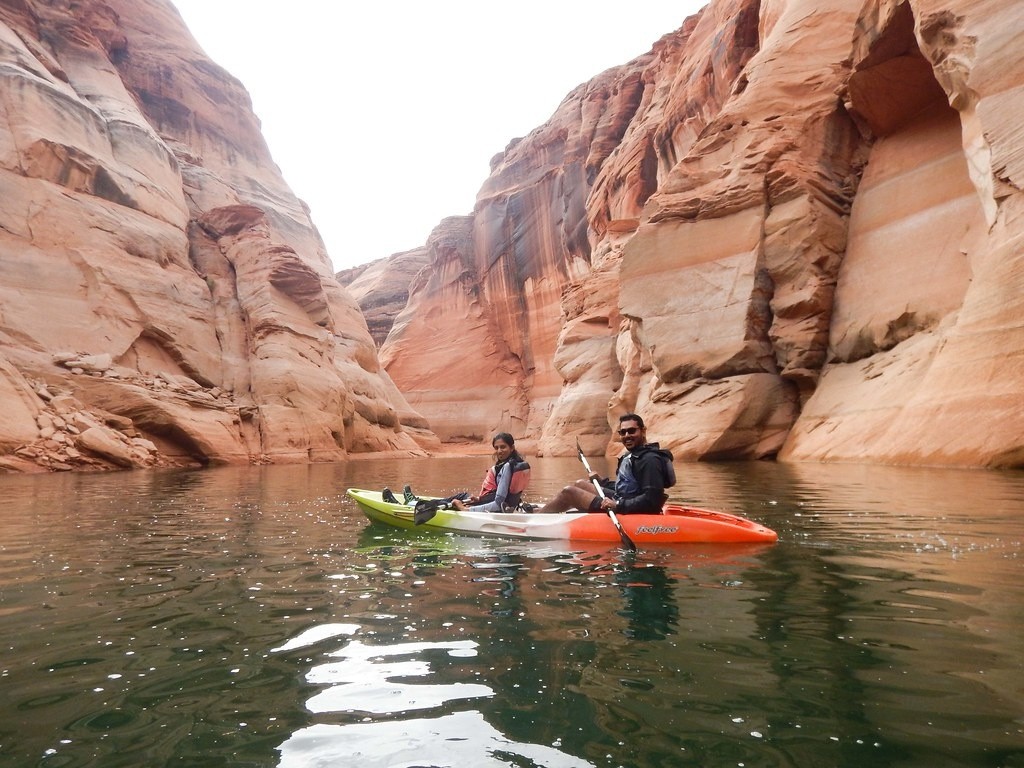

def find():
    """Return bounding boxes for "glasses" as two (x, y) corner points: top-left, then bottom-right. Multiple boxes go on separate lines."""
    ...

(618, 427), (641, 436)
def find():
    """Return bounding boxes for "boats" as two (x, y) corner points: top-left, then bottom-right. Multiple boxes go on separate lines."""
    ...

(346, 487), (778, 544)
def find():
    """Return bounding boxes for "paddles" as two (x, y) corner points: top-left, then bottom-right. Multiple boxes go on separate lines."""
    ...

(413, 492), (483, 524)
(574, 440), (635, 552)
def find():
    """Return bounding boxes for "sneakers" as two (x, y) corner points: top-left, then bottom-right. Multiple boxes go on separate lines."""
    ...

(403, 484), (419, 504)
(500, 502), (533, 514)
(382, 488), (401, 506)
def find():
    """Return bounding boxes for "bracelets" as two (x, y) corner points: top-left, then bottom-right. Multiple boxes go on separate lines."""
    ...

(616, 500), (620, 512)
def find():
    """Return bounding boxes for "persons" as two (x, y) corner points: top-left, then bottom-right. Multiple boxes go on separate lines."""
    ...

(532, 413), (677, 515)
(382, 432), (531, 512)
